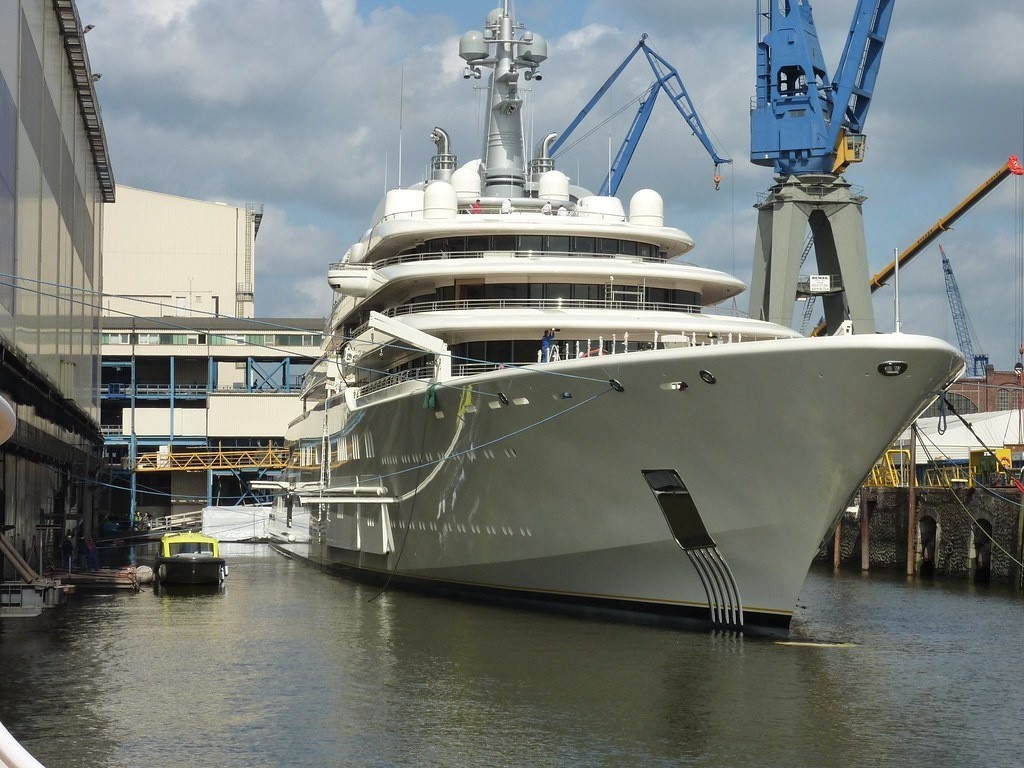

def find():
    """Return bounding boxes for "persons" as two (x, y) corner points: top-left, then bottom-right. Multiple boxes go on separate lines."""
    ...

(540, 201), (568, 216)
(542, 329), (554, 362)
(62, 534), (99, 574)
(501, 198), (512, 214)
(125, 510), (149, 533)
(472, 199), (481, 213)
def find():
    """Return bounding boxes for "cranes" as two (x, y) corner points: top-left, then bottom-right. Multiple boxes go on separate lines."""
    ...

(751, 0), (896, 336)
(939, 247), (991, 379)
(546, 44), (737, 198)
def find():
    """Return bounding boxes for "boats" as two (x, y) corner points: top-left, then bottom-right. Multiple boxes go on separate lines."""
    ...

(148, 533), (227, 600)
(265, 2), (964, 643)
